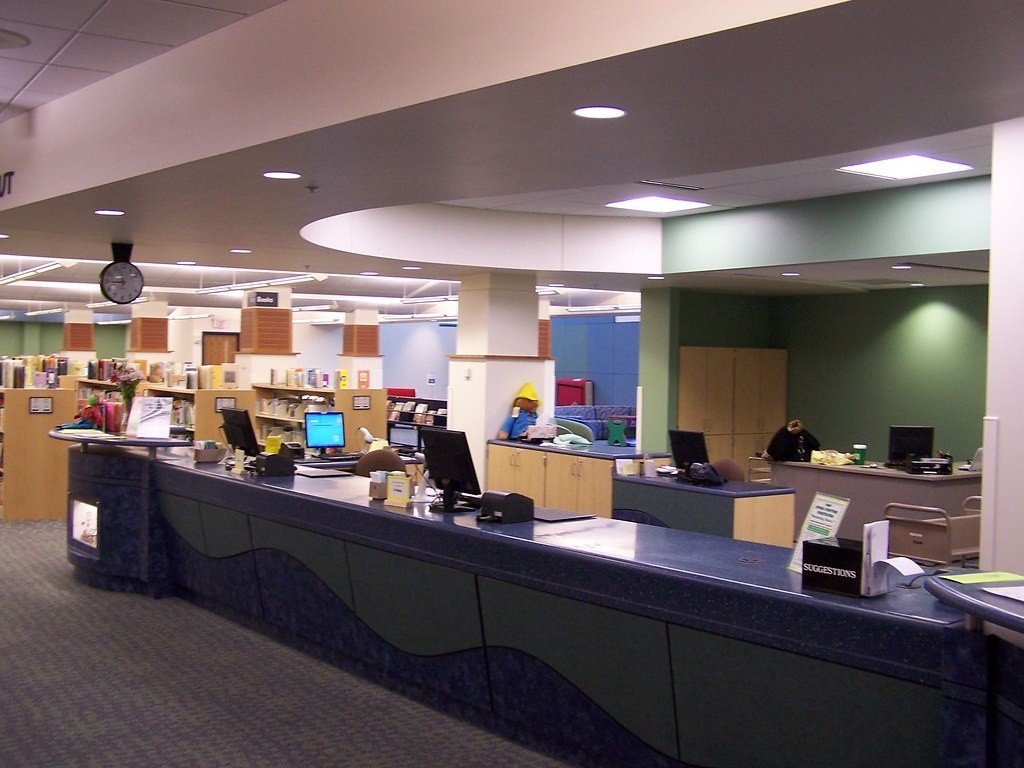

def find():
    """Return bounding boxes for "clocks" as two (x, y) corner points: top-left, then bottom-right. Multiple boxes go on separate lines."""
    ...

(100, 243), (144, 304)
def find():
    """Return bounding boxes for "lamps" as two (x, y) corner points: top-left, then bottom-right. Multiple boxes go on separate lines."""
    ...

(401, 279), (459, 304)
(172, 314), (215, 320)
(194, 264), (329, 295)
(566, 292), (641, 312)
(383, 305), (459, 320)
(292, 318), (340, 324)
(85, 293), (149, 309)
(292, 301), (339, 312)
(24, 301), (70, 317)
(0, 255), (76, 285)
(0, 310), (16, 322)
(535, 286), (562, 297)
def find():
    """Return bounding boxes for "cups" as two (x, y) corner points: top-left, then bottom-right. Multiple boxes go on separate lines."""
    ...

(853, 444), (867, 465)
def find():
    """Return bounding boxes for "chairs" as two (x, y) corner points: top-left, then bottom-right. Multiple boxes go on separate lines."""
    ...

(555, 378), (595, 407)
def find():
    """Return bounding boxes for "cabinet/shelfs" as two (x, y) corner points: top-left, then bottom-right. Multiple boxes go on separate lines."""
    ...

(57, 374), (86, 388)
(252, 382), (388, 452)
(389, 396), (447, 429)
(0, 388), (78, 522)
(78, 378), (144, 438)
(143, 386), (258, 446)
(545, 452), (672, 519)
(882, 495), (981, 569)
(487, 444), (545, 507)
(679, 344), (789, 474)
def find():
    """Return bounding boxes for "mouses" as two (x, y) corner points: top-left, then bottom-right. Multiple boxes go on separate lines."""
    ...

(870, 463), (877, 468)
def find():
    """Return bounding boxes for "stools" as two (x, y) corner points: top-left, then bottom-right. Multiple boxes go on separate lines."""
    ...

(606, 414), (636, 447)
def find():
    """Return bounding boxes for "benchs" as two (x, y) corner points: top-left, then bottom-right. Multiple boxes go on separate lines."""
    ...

(555, 405), (636, 440)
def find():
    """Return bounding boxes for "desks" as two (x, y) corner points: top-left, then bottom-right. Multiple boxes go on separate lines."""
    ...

(49, 426), (193, 601)
(770, 460), (982, 549)
(927, 570), (1024, 768)
(611, 474), (795, 549)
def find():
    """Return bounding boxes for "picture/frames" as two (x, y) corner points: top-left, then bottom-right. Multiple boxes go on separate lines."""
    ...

(215, 396), (237, 413)
(352, 395), (371, 410)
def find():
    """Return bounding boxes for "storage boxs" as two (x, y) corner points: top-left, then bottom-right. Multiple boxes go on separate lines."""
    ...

(357, 370), (369, 388)
(106, 401), (124, 433)
(195, 448), (227, 463)
(335, 369), (349, 388)
(220, 362), (239, 390)
(384, 473), (417, 508)
(35, 373), (47, 385)
(287, 368), (296, 386)
(265, 435), (281, 454)
(47, 367), (59, 387)
(291, 430), (305, 443)
(801, 536), (863, 598)
(369, 481), (387, 500)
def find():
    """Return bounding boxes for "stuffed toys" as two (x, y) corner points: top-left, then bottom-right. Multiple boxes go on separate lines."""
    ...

(499, 382), (541, 441)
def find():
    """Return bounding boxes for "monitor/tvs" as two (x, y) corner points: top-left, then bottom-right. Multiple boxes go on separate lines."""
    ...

(669, 429), (709, 470)
(304, 412), (345, 457)
(888, 424), (934, 465)
(419, 426), (482, 512)
(221, 407), (260, 470)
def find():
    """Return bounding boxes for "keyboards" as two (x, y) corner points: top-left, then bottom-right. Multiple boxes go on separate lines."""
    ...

(885, 461), (905, 468)
(459, 493), (482, 509)
(322, 453), (362, 461)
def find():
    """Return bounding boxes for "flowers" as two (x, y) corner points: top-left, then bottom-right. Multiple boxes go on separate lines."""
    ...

(109, 365), (144, 412)
